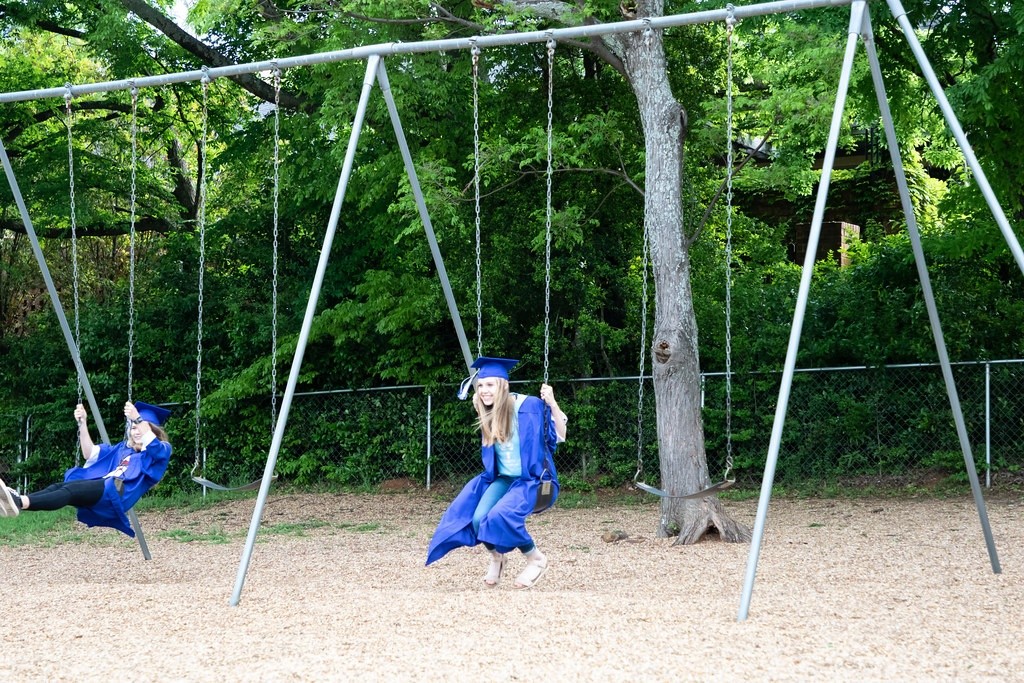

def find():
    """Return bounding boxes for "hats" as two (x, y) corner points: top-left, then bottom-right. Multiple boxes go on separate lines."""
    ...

(471, 356), (519, 380)
(134, 401), (171, 425)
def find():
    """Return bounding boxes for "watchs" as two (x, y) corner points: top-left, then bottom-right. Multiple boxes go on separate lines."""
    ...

(133, 416), (143, 424)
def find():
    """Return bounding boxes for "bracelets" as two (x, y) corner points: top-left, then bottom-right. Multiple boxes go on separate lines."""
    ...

(550, 401), (557, 408)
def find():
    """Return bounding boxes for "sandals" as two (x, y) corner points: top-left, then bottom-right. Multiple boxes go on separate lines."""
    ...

(510, 561), (549, 590)
(482, 553), (508, 589)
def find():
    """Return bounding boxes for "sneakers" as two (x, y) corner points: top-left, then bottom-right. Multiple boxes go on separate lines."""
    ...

(0, 478), (23, 518)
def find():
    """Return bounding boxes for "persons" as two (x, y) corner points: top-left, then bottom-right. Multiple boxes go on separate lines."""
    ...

(0, 401), (173, 538)
(426, 358), (568, 591)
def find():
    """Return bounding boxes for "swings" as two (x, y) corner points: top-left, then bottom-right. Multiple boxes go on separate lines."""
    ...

(190, 70), (280, 491)
(470, 40), (558, 515)
(632, 21), (738, 502)
(64, 89), (138, 515)
